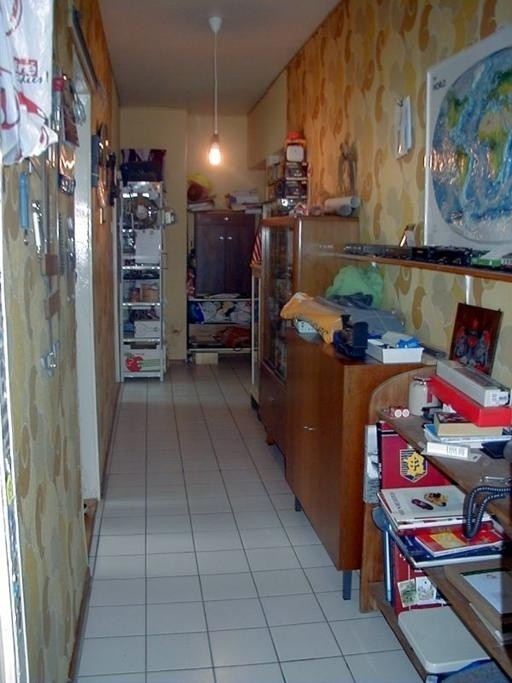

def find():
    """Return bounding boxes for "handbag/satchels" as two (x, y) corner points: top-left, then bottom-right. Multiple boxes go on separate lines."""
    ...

(214, 327), (253, 347)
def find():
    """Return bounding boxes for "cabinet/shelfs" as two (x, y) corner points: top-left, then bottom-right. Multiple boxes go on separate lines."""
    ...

(357, 363), (510, 682)
(249, 213), (361, 428)
(180, 204), (268, 364)
(271, 324), (438, 603)
(110, 179), (169, 384)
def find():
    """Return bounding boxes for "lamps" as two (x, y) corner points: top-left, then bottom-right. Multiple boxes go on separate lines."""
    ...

(205, 14), (224, 167)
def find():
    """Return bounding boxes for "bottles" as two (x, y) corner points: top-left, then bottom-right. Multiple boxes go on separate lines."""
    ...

(407, 375), (439, 416)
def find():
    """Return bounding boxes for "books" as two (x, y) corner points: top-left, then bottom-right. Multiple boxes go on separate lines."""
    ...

(372, 422), (511, 652)
